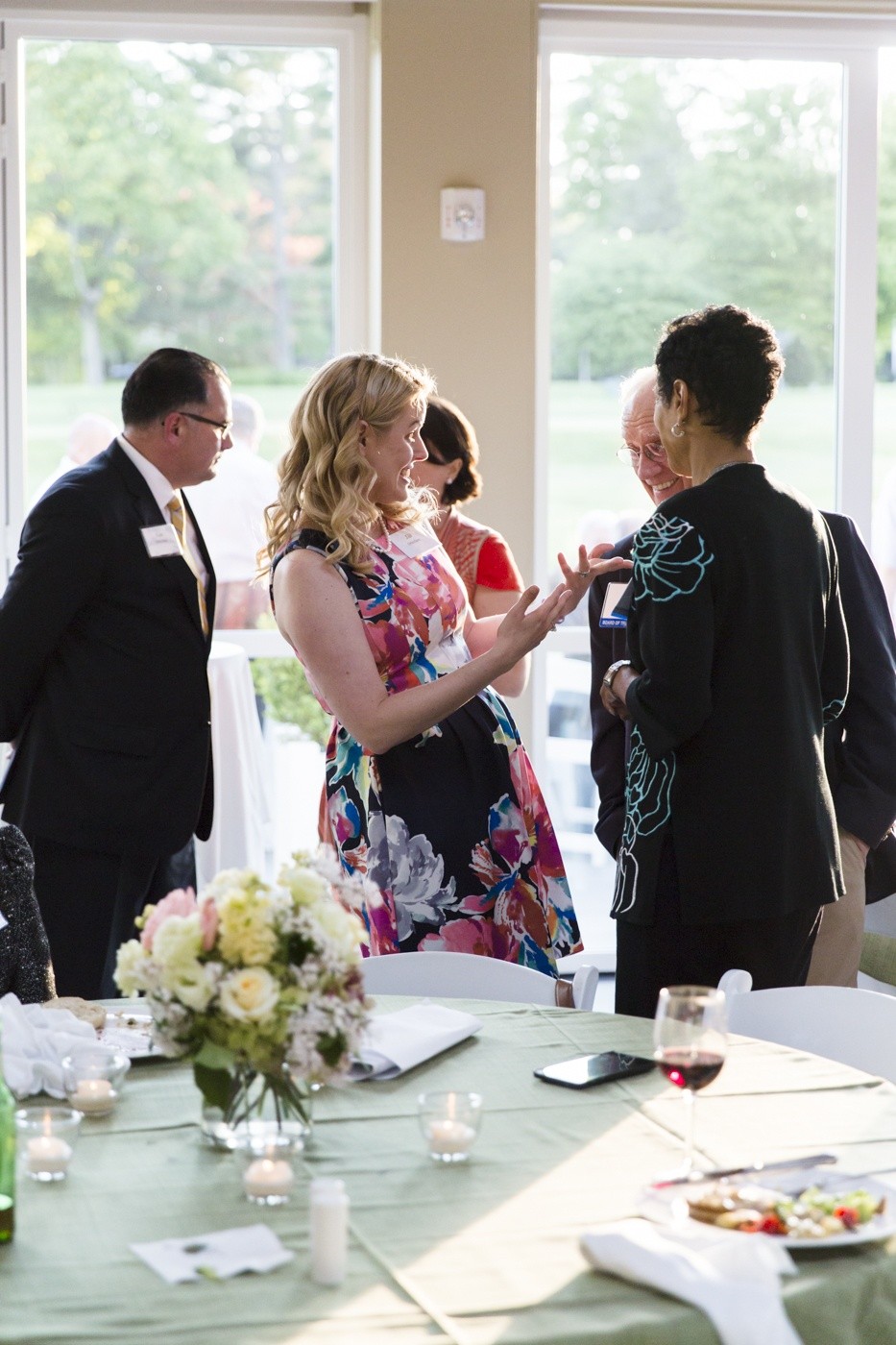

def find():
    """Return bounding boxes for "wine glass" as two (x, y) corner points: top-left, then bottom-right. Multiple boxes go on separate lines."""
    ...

(652, 986), (729, 1172)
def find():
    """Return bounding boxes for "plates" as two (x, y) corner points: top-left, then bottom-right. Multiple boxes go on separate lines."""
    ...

(96, 1004), (164, 1058)
(669, 1166), (895, 1249)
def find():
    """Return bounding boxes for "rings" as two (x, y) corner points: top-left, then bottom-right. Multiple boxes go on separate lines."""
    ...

(550, 622), (556, 631)
(578, 569), (590, 577)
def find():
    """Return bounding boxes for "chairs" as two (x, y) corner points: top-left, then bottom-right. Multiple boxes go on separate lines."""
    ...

(705, 969), (895, 1085)
(359, 949), (599, 1011)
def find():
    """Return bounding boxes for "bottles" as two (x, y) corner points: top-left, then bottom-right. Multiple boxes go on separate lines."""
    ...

(1, 1072), (17, 1244)
(305, 1181), (349, 1285)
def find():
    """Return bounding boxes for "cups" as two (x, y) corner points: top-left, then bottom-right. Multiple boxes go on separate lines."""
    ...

(232, 1132), (305, 1208)
(60, 1053), (130, 1116)
(15, 1106), (84, 1183)
(418, 1092), (484, 1161)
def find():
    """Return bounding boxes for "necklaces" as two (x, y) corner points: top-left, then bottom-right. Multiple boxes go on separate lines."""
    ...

(360, 512), (392, 552)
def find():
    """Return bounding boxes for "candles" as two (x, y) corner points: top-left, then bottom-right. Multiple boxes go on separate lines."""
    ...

(15, 1107), (84, 1183)
(229, 1131), (298, 1204)
(62, 1051), (133, 1117)
(417, 1088), (483, 1167)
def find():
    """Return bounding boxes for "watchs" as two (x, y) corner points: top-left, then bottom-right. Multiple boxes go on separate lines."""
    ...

(599, 660), (631, 717)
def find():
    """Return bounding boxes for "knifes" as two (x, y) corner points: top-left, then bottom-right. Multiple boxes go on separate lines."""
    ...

(652, 1153), (838, 1188)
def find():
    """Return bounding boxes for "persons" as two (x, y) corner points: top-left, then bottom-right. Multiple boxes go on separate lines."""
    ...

(588, 367), (896, 988)
(0, 348), (231, 1000)
(598, 305), (849, 1019)
(250, 353), (636, 980)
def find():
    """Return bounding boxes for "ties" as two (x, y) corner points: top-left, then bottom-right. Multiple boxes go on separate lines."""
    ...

(169, 493), (209, 640)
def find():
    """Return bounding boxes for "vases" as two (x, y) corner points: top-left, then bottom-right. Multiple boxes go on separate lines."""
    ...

(190, 1046), (318, 1156)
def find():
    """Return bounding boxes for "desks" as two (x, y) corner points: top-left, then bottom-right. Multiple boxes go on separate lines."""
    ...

(0, 996), (896, 1345)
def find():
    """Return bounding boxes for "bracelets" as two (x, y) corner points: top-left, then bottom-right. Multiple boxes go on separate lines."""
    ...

(542, 599), (565, 624)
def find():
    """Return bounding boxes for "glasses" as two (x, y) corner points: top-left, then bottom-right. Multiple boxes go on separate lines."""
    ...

(616, 441), (667, 468)
(162, 412), (233, 440)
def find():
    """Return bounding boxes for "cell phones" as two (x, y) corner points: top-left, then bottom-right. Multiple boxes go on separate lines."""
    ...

(532, 1050), (656, 1090)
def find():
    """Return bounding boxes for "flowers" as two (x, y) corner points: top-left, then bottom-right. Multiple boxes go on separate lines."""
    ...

(107, 840), (379, 1102)
